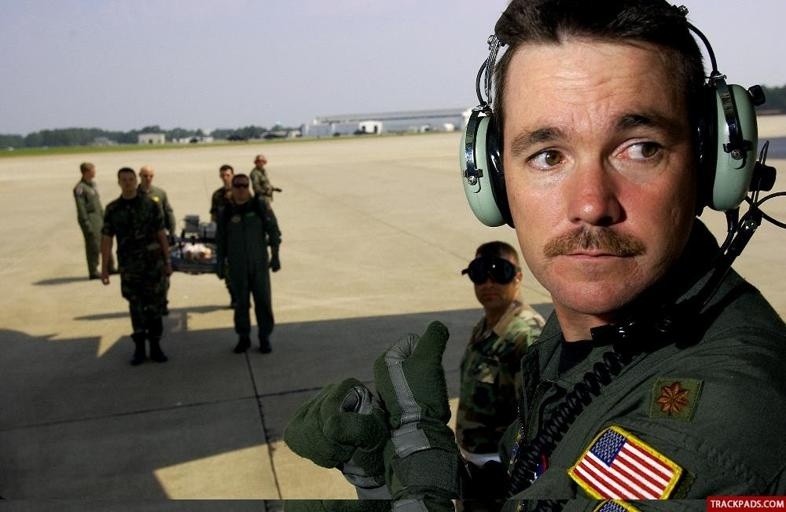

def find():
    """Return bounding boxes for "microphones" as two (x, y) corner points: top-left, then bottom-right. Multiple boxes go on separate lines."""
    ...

(590, 210), (740, 346)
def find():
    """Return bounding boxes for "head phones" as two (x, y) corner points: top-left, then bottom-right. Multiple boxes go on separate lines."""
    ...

(459, 17), (757, 226)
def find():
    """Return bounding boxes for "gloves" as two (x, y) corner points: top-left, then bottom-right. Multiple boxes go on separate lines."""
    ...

(268, 252), (281, 273)
(281, 376), (393, 491)
(372, 318), (465, 503)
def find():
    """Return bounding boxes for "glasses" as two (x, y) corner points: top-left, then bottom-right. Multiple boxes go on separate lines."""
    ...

(232, 182), (249, 188)
(462, 258), (523, 287)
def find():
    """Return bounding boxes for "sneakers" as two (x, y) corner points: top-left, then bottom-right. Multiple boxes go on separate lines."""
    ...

(106, 267), (120, 275)
(130, 345), (147, 366)
(231, 334), (252, 355)
(89, 273), (103, 280)
(257, 332), (273, 355)
(149, 345), (169, 363)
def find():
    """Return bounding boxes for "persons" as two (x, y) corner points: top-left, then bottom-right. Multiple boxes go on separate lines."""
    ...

(72, 162), (117, 279)
(136, 164), (175, 248)
(214, 173), (283, 355)
(249, 153), (282, 246)
(452, 240), (548, 512)
(208, 163), (252, 310)
(98, 168), (174, 366)
(183, 243), (211, 263)
(281, 0), (786, 512)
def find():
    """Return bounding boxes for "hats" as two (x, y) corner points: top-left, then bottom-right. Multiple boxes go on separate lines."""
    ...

(253, 154), (268, 165)
